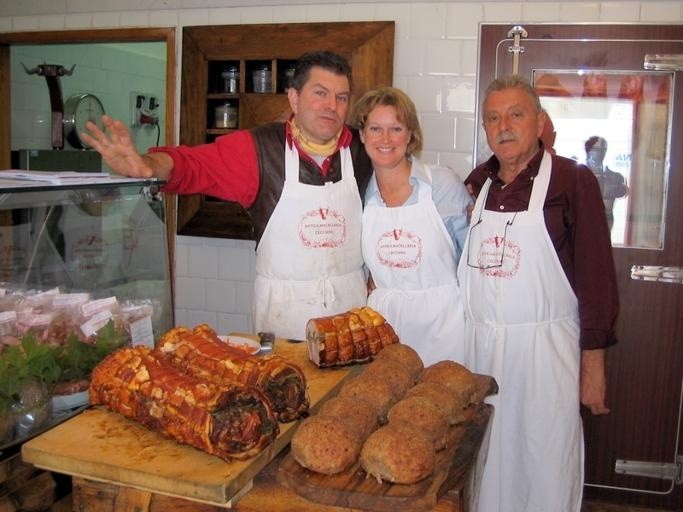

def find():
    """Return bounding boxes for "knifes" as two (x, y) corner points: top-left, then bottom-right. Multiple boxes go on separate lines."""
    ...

(258, 325), (275, 358)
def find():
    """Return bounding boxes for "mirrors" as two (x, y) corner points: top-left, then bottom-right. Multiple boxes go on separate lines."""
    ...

(525, 68), (674, 251)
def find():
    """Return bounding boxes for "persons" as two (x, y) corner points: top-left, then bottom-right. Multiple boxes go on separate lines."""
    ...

(574, 132), (630, 233)
(347, 80), (477, 373)
(452, 71), (624, 512)
(77, 48), (374, 345)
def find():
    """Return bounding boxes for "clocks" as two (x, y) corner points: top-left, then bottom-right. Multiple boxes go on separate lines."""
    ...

(62, 94), (106, 150)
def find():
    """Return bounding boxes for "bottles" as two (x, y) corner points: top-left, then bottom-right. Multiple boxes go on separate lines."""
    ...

(221, 66), (239, 93)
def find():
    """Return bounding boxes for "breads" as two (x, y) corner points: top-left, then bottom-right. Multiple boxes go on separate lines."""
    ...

(89, 306), (476, 486)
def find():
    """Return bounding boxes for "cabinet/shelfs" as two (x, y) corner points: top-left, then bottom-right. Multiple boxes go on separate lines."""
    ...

(1, 167), (175, 499)
(176, 20), (395, 243)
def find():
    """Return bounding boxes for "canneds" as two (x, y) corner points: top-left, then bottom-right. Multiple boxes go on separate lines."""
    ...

(283, 63), (298, 94)
(220, 65), (240, 94)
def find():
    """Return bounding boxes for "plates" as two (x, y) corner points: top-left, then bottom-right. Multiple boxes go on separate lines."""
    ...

(214, 330), (260, 354)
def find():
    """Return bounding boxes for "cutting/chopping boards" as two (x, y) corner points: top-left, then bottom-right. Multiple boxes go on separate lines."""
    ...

(276, 371), (500, 510)
(21, 331), (360, 504)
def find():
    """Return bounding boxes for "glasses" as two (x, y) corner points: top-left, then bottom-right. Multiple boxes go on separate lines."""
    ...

(464, 219), (511, 269)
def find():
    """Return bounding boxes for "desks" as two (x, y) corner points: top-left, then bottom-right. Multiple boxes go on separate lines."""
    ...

(21, 331), (494, 512)
